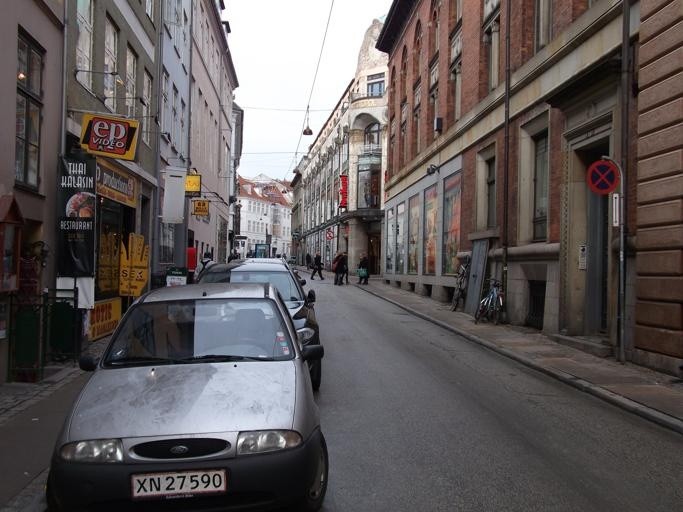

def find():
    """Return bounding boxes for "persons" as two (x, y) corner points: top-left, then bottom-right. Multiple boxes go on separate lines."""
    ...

(187, 239), (198, 283)
(307, 252), (368, 286)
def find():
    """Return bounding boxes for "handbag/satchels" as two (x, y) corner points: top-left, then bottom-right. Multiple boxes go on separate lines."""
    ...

(358, 269), (366, 277)
(313, 266), (318, 269)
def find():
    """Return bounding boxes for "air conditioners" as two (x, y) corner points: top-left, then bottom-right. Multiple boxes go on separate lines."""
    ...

(434, 118), (442, 133)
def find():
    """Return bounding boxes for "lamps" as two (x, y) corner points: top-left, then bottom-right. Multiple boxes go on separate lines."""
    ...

(427, 164), (439, 174)
(75, 70), (228, 206)
(303, 106), (313, 135)
(292, 152), (299, 173)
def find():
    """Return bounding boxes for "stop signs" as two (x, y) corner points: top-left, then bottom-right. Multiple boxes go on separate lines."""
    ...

(588, 159), (620, 194)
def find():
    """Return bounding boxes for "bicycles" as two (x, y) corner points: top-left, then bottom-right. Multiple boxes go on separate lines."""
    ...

(451, 256), (471, 311)
(474, 278), (504, 325)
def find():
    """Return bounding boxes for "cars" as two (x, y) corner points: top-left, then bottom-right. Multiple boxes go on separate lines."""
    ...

(45, 281), (328, 511)
(195, 255), (328, 391)
